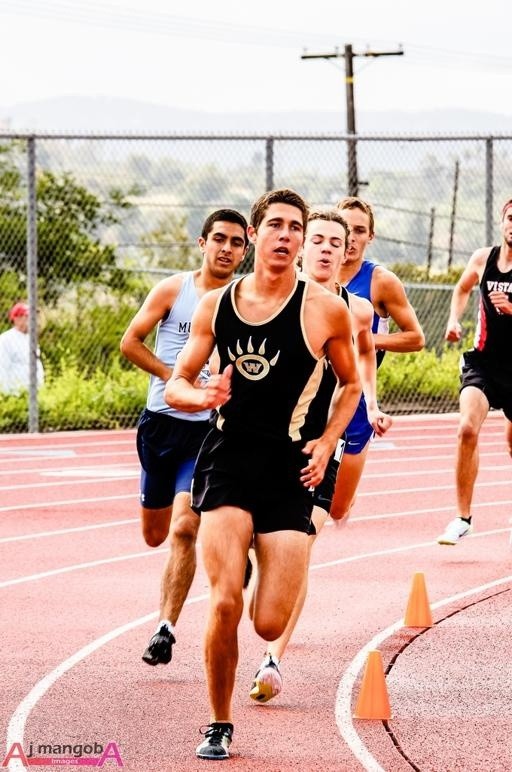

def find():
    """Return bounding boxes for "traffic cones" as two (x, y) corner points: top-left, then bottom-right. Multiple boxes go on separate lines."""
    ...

(403, 571), (433, 627)
(353, 648), (396, 722)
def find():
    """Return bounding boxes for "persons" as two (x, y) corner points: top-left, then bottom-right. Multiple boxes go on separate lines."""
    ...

(119, 188), (427, 759)
(0, 302), (44, 397)
(437, 198), (512, 545)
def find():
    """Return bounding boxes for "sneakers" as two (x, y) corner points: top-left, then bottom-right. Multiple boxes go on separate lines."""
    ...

(250, 664), (284, 703)
(142, 632), (176, 666)
(437, 516), (474, 545)
(195, 721), (235, 758)
